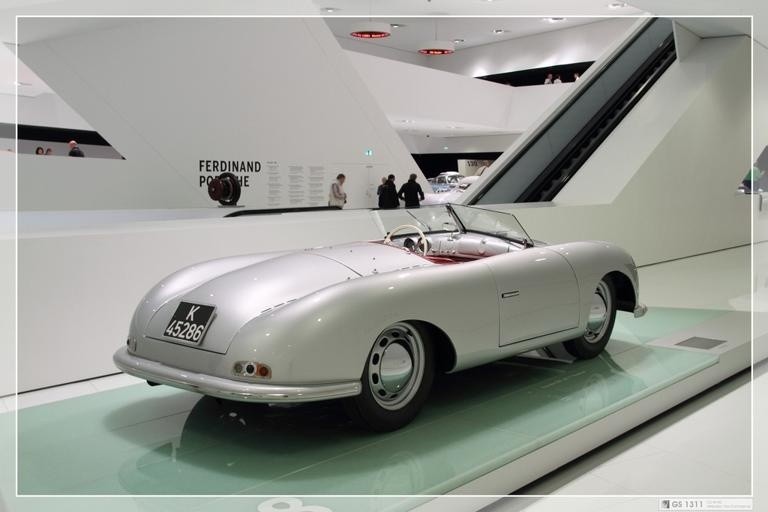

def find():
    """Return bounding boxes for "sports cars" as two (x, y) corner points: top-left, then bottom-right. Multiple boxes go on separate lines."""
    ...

(111, 202), (648, 433)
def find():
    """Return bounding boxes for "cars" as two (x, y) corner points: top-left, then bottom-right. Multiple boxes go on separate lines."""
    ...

(428, 171), (465, 193)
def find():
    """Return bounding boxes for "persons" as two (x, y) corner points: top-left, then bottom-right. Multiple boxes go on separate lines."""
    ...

(743, 162), (766, 192)
(573, 72), (580, 82)
(36, 147), (52, 155)
(328, 174), (346, 209)
(68, 140), (84, 157)
(544, 73), (562, 84)
(377, 174), (424, 208)
